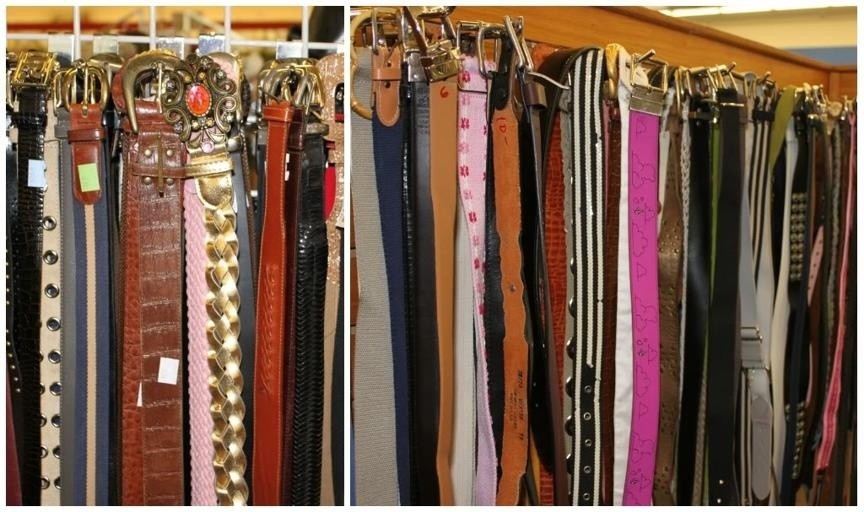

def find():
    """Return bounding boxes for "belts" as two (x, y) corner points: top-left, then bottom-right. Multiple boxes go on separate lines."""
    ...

(351, 8), (409, 505)
(457, 14), (568, 506)
(123, 50), (190, 505)
(292, 53), (345, 504)
(818, 99), (856, 507)
(412, 7), (457, 505)
(537, 43), (668, 506)
(250, 50), (312, 506)
(770, 81), (828, 505)
(188, 52), (256, 506)
(6, 50), (60, 506)
(652, 65), (744, 506)
(54, 52), (123, 507)
(738, 74), (776, 505)
